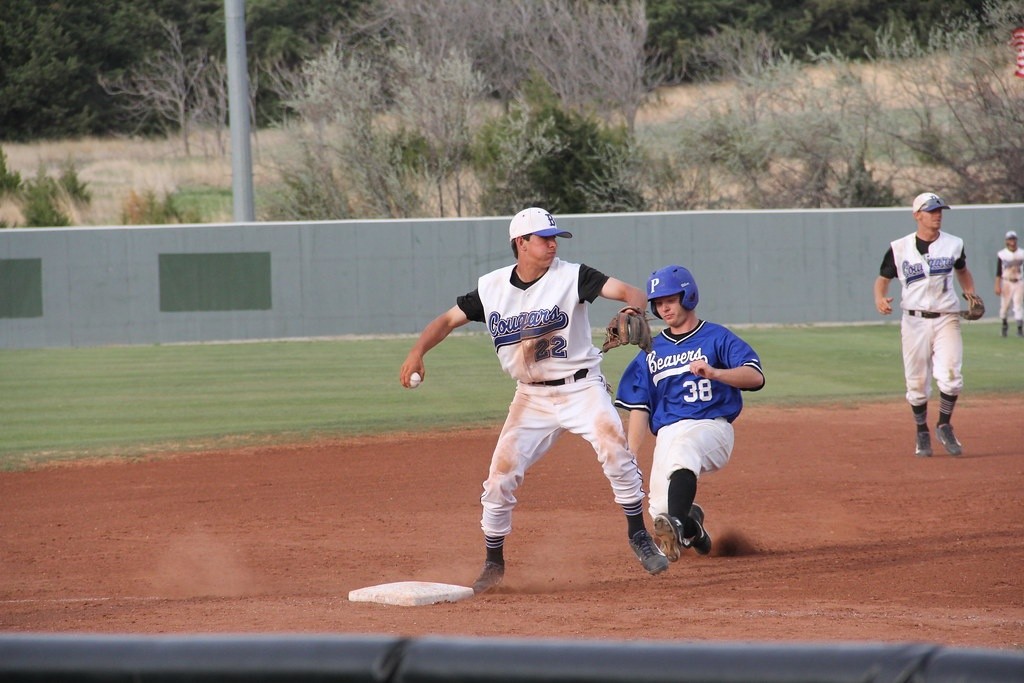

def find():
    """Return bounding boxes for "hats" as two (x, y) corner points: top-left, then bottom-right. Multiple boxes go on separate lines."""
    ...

(510, 207), (572, 241)
(1005, 231), (1017, 239)
(913, 192), (951, 213)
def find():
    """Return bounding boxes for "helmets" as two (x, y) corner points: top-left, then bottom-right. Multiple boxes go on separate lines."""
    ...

(647, 264), (698, 319)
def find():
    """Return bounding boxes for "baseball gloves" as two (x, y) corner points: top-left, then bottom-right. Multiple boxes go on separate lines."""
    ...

(960, 292), (986, 320)
(602, 306), (652, 355)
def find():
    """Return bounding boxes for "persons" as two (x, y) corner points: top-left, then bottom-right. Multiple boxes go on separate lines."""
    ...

(995, 230), (1024, 337)
(874, 193), (985, 456)
(615, 264), (765, 561)
(400, 207), (669, 593)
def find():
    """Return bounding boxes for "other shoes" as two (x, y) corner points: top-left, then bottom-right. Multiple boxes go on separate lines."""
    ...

(1002, 328), (1008, 336)
(1017, 332), (1024, 337)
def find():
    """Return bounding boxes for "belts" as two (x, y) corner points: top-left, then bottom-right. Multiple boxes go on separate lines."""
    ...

(909, 310), (940, 318)
(533, 369), (588, 386)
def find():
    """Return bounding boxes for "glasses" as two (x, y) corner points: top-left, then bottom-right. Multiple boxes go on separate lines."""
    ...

(917, 198), (945, 213)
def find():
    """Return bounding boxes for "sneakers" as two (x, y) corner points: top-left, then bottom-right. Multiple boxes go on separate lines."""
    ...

(653, 513), (684, 562)
(689, 503), (712, 556)
(915, 429), (932, 456)
(471, 560), (505, 595)
(628, 530), (669, 574)
(935, 422), (961, 455)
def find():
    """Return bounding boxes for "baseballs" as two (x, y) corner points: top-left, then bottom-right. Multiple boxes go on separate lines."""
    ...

(409, 372), (421, 388)
